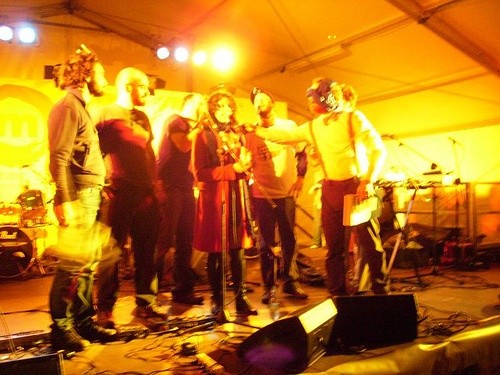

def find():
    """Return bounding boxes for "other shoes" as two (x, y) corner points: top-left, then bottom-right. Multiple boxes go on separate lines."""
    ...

(97, 310), (122, 327)
(138, 301), (169, 319)
(211, 303), (225, 323)
(282, 280), (308, 298)
(261, 285), (275, 304)
(235, 296), (258, 314)
(170, 290), (206, 304)
(47, 318), (115, 351)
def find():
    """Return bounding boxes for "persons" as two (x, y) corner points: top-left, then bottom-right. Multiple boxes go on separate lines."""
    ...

(241, 87), (309, 304)
(154, 94), (209, 304)
(195, 91), (258, 322)
(94, 67), (168, 329)
(49, 50), (116, 350)
(237, 77), (390, 295)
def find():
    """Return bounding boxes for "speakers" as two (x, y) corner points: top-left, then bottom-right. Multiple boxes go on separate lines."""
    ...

(0, 352), (65, 375)
(233, 297), (338, 375)
(324, 292), (417, 355)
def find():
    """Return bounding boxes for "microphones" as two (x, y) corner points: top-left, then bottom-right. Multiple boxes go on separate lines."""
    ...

(205, 114), (217, 130)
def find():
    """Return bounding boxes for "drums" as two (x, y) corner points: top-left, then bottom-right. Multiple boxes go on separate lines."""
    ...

(0, 226), (36, 278)
(17, 189), (48, 220)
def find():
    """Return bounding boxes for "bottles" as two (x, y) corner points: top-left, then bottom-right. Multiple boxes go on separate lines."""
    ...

(269, 290), (280, 320)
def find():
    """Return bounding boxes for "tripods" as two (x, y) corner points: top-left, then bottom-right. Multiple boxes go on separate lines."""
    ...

(169, 120), (278, 339)
(397, 187), (465, 284)
